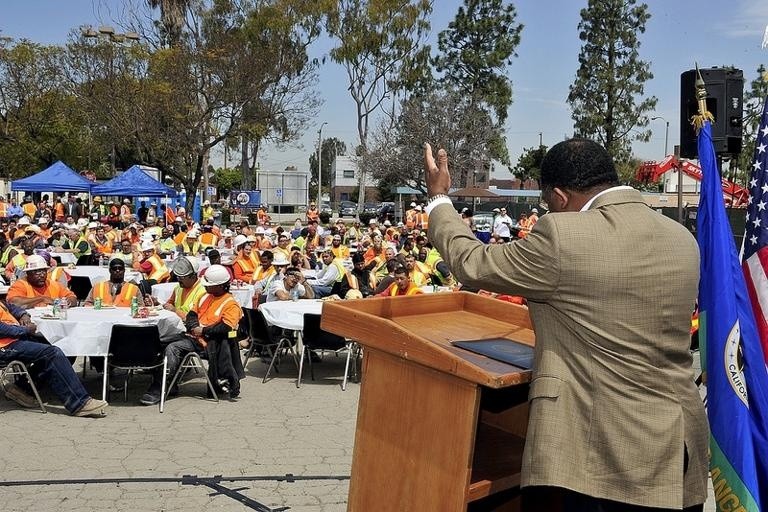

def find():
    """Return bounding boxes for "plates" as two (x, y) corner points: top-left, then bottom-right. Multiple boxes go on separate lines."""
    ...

(101, 305), (116, 310)
(38, 315), (60, 320)
(146, 305), (164, 316)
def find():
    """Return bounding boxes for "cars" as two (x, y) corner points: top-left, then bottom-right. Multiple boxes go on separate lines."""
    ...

(469, 210), (494, 225)
(320, 198), (397, 219)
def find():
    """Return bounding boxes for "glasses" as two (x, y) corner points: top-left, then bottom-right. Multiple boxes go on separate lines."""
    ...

(121, 245), (130, 248)
(111, 266), (123, 271)
(34, 270), (47, 275)
(288, 272), (294, 275)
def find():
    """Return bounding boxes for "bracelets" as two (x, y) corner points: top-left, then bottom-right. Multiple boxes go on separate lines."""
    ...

(427, 195), (449, 207)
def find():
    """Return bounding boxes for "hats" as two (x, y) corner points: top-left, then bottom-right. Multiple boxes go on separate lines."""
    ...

(175, 203), (180, 208)
(352, 254), (364, 265)
(150, 202), (156, 206)
(202, 200), (210, 206)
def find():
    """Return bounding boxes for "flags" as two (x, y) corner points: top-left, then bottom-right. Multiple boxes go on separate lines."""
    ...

(738, 90), (768, 373)
(695, 70), (768, 510)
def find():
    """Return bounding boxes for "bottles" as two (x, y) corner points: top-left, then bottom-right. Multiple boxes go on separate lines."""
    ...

(315, 264), (319, 275)
(58, 296), (68, 320)
(10, 272), (17, 286)
(98, 255), (103, 268)
(292, 285), (299, 302)
(105, 256), (108, 268)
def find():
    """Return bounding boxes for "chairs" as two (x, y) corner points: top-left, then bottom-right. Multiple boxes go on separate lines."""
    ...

(1, 349), (46, 414)
(101, 324), (168, 414)
(240, 306), (298, 384)
(296, 313), (358, 391)
(165, 350), (221, 404)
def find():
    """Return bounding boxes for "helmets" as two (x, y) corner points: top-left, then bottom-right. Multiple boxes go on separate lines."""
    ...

(344, 289), (362, 300)
(87, 221), (98, 228)
(91, 197), (138, 219)
(270, 252), (290, 266)
(200, 264), (230, 287)
(168, 256), (199, 277)
(139, 241), (154, 252)
(19, 217), (52, 236)
(368, 217), (404, 228)
(22, 255), (50, 272)
(325, 220), (345, 241)
(25, 196), (32, 202)
(311, 201), (316, 205)
(168, 217), (201, 239)
(220, 226), (264, 246)
(407, 203), (421, 229)
(77, 218), (89, 227)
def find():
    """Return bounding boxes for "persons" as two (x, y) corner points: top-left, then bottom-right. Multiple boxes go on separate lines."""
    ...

(2, 193), (245, 416)
(405, 201), (429, 234)
(423, 137), (714, 511)
(257, 203), (272, 226)
(306, 202), (320, 225)
(378, 203), (393, 223)
(460, 206), (540, 245)
(233, 221), (456, 364)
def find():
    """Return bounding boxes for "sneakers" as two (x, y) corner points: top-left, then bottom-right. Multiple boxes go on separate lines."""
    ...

(139, 389), (161, 405)
(74, 398), (108, 417)
(4, 383), (40, 408)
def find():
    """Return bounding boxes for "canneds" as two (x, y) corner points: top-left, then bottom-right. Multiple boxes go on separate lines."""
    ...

(433, 285), (439, 293)
(94, 297), (101, 310)
(53, 298), (61, 312)
(292, 291), (299, 301)
(131, 303), (138, 316)
(132, 297), (138, 303)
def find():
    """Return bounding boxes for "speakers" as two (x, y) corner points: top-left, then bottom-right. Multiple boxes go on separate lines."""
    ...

(680, 68), (744, 158)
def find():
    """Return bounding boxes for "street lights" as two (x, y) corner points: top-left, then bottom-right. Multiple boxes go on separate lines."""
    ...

(317, 121), (329, 213)
(650, 115), (670, 158)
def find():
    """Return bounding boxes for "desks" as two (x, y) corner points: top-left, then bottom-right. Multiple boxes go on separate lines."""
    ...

(23, 305), (176, 402)
(257, 299), (324, 381)
(1, 247), (358, 316)
